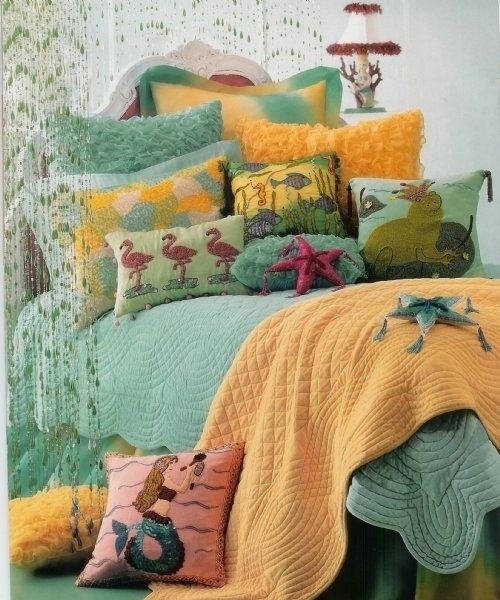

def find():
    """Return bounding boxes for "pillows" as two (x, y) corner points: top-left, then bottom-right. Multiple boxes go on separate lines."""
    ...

(69, 435), (253, 593)
(37, 99), (225, 176)
(105, 214), (248, 318)
(136, 65), (346, 132)
(229, 229), (363, 291)
(216, 148), (349, 239)
(344, 169), (493, 280)
(237, 108), (429, 210)
(19, 138), (246, 330)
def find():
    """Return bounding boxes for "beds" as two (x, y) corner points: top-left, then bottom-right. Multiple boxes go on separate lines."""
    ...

(4, 35), (498, 598)
